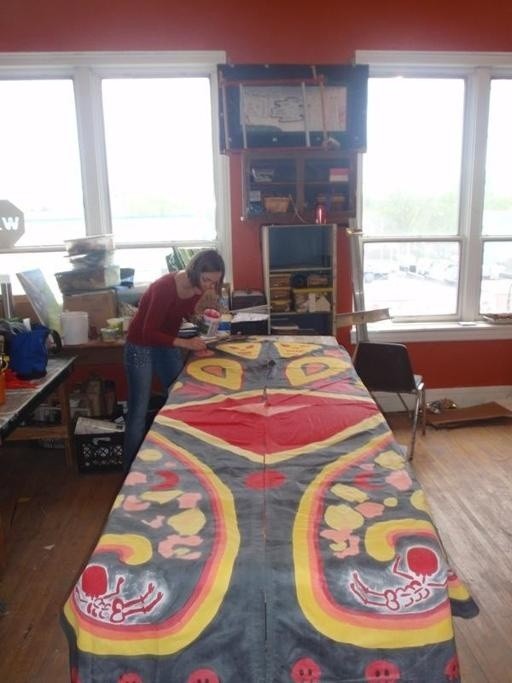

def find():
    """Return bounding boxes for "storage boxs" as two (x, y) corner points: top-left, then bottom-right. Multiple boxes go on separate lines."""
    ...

(54, 234), (119, 337)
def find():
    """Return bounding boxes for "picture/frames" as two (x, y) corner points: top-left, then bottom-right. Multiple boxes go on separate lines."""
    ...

(216, 63), (369, 154)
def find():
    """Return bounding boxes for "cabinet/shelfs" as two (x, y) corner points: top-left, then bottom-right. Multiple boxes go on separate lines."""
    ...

(240, 149), (359, 339)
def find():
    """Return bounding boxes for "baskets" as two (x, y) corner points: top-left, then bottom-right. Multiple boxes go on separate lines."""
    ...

(74, 414), (153, 474)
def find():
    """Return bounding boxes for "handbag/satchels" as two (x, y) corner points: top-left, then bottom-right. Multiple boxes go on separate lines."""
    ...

(0, 317), (51, 380)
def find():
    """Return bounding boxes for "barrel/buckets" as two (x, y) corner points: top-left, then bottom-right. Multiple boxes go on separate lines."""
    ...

(59, 310), (89, 347)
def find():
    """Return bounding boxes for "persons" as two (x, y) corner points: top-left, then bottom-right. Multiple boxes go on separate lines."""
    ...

(121, 249), (225, 480)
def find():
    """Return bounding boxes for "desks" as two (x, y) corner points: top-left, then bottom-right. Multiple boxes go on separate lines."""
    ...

(59, 333), (479, 683)
(61, 335), (193, 366)
(1, 346), (80, 478)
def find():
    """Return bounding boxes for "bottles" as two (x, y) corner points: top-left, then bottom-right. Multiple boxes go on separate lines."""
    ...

(217, 287), (229, 313)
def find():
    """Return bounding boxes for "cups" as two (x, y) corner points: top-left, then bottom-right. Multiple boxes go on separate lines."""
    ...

(106, 317), (122, 336)
(101, 328), (119, 342)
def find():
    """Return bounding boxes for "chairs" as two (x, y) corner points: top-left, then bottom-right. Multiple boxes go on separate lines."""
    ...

(349, 340), (427, 463)
